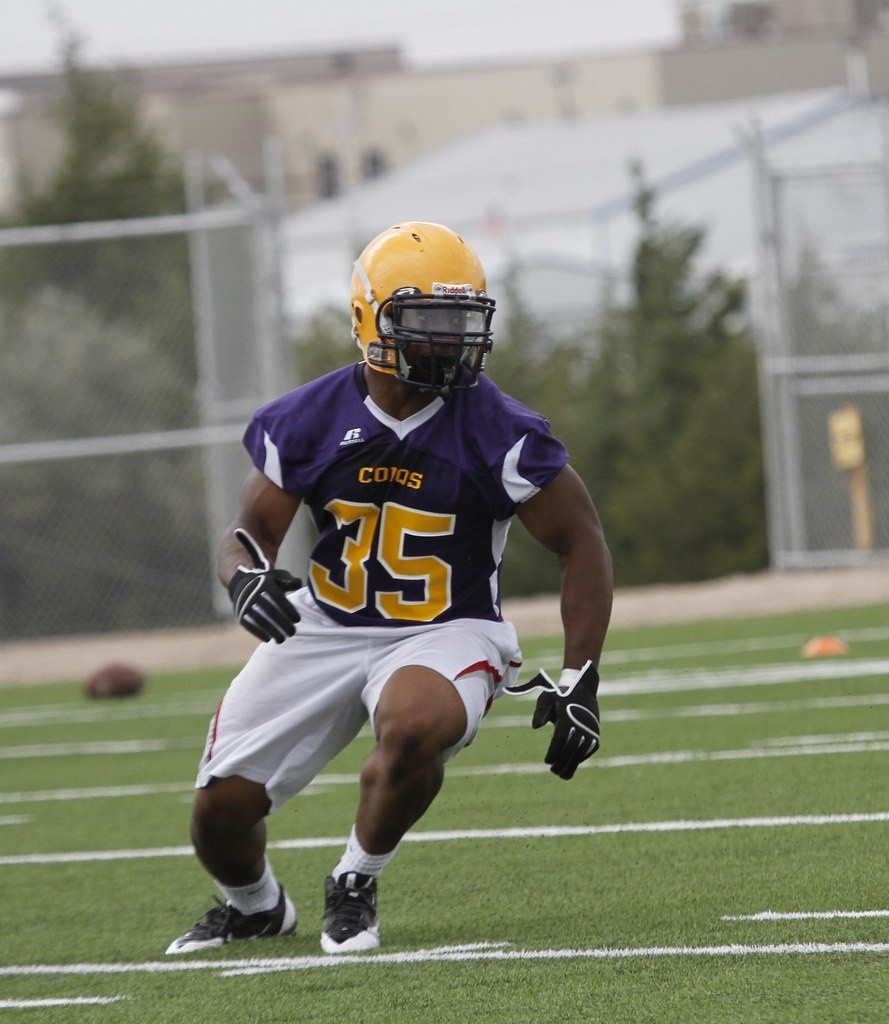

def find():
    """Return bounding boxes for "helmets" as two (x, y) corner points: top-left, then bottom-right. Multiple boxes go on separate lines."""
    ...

(350, 222), (498, 395)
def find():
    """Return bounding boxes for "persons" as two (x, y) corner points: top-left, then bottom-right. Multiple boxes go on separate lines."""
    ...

(167, 221), (614, 954)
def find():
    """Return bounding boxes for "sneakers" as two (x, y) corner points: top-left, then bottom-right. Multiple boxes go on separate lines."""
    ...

(320, 870), (381, 954)
(166, 884), (296, 955)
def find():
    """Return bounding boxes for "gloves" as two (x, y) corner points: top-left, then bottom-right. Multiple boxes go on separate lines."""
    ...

(228, 528), (302, 644)
(501, 659), (600, 781)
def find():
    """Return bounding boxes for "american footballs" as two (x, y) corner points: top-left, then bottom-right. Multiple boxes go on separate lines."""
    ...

(83, 665), (144, 699)
